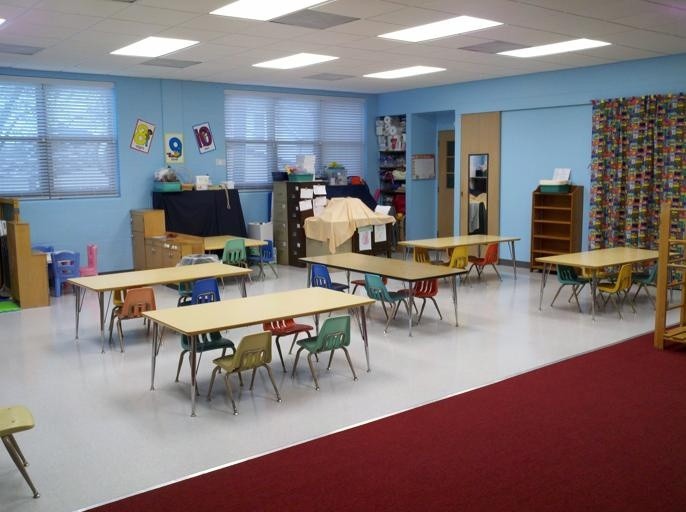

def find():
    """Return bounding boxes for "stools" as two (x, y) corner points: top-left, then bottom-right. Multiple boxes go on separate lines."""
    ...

(0, 402), (42, 499)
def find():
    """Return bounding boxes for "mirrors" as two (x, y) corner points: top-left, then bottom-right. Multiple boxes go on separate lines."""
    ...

(467, 152), (489, 235)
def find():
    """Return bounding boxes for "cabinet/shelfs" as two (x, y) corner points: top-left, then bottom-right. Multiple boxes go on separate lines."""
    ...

(150, 174), (378, 267)
(527, 178), (585, 279)
(302, 215), (396, 274)
(376, 146), (407, 197)
(127, 207), (204, 296)
(0, 196), (52, 312)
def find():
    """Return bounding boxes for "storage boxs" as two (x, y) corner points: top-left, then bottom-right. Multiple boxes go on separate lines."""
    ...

(269, 166), (347, 186)
(537, 183), (570, 195)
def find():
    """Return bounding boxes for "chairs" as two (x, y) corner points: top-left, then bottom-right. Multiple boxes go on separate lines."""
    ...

(286, 314), (359, 395)
(111, 287), (162, 354)
(175, 279), (201, 307)
(244, 238), (281, 281)
(29, 239), (101, 300)
(413, 242), (502, 284)
(104, 283), (152, 347)
(177, 275), (220, 307)
(550, 265), (659, 320)
(204, 329), (283, 418)
(262, 317), (319, 376)
(173, 328), (243, 399)
(216, 239), (254, 289)
(307, 262), (442, 332)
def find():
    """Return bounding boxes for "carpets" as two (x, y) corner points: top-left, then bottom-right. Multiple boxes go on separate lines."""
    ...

(71, 323), (686, 512)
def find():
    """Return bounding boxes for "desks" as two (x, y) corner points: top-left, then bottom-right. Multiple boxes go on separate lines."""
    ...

(199, 233), (269, 284)
(65, 260), (254, 355)
(398, 236), (520, 284)
(135, 284), (378, 425)
(300, 252), (468, 334)
(535, 247), (677, 320)
